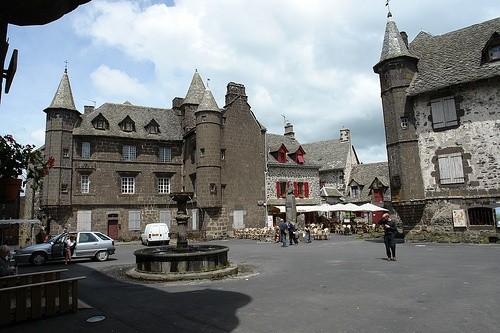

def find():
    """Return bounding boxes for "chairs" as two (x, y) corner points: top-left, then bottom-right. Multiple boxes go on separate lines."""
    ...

(233, 220), (384, 240)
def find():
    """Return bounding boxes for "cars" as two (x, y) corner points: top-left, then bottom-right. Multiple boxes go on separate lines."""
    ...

(15, 230), (116, 265)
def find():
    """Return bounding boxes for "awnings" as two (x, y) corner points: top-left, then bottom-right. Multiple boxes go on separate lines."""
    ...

(272, 205), (320, 214)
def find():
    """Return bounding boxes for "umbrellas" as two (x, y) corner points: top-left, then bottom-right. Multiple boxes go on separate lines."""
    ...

(314, 203), (390, 226)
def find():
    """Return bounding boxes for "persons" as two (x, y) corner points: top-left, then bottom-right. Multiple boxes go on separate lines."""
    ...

(273, 213), (374, 247)
(378, 212), (397, 261)
(36, 225), (47, 244)
(64, 234), (77, 265)
(0, 245), (17, 277)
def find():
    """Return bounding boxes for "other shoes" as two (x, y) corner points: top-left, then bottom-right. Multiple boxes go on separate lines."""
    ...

(393, 257), (397, 260)
(388, 258), (391, 261)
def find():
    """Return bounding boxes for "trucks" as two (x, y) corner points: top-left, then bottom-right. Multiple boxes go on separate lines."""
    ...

(141, 222), (170, 244)
